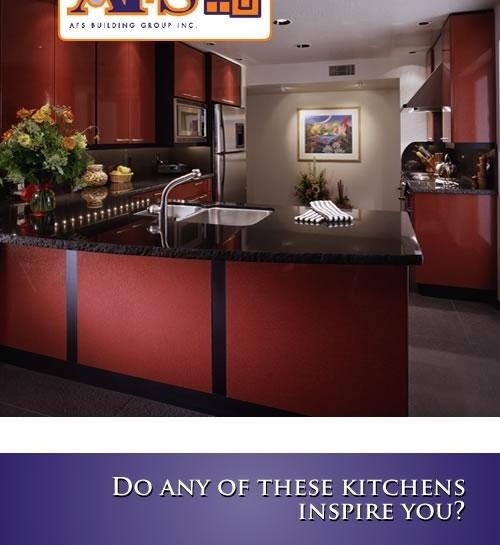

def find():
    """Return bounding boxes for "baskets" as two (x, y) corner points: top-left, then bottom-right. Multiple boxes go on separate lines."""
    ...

(109, 173), (133, 183)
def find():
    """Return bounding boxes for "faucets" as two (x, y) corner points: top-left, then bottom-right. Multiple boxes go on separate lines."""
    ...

(154, 168), (204, 229)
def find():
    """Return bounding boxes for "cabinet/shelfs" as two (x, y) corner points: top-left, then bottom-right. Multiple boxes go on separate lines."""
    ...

(156, 41), (206, 102)
(208, 53), (241, 107)
(139, 178), (212, 206)
(0, 1), (95, 144)
(95, 39), (155, 143)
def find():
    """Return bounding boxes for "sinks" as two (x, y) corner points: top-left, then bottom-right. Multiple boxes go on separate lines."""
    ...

(148, 202), (201, 223)
(181, 205), (273, 228)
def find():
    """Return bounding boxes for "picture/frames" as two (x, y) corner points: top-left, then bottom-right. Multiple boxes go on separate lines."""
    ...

(296, 106), (360, 162)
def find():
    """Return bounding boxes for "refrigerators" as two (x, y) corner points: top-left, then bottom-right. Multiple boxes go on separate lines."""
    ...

(212, 103), (248, 206)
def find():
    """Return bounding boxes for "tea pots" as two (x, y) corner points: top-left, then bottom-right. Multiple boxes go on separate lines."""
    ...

(438, 154), (456, 178)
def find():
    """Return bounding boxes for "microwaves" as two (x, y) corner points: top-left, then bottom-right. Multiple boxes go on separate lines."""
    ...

(173, 98), (207, 144)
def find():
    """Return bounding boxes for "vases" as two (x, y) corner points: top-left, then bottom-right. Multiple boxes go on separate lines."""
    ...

(31, 184), (55, 212)
(81, 164), (109, 188)
(80, 187), (109, 209)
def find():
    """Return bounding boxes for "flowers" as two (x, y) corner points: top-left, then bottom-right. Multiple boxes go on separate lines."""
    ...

(0, 106), (96, 183)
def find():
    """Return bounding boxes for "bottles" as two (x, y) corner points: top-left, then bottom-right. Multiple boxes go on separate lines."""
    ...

(478, 154), (489, 189)
(416, 151), (426, 163)
(419, 147), (432, 158)
